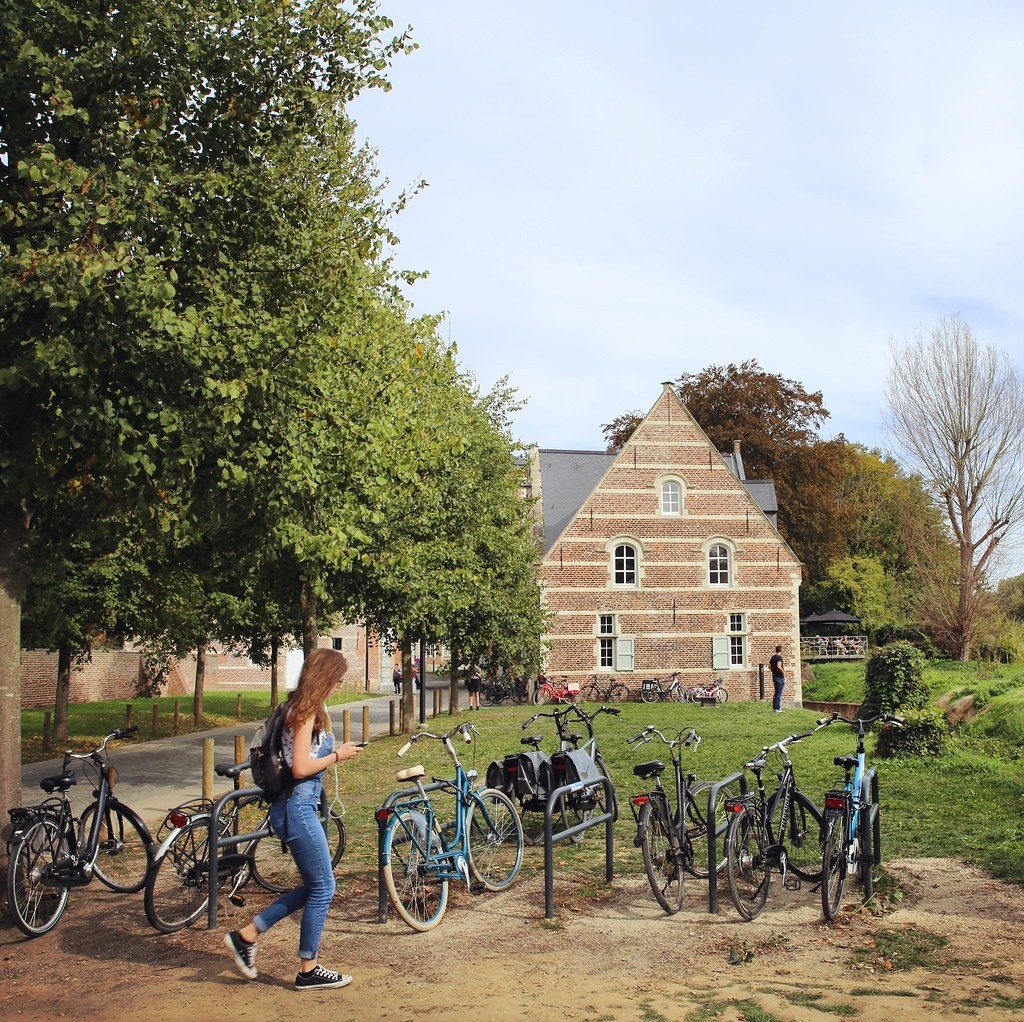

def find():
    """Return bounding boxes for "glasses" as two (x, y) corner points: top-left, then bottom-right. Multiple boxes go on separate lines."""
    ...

(338, 679), (343, 686)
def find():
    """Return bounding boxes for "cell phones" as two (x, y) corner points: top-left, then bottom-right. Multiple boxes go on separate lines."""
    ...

(354, 741), (368, 747)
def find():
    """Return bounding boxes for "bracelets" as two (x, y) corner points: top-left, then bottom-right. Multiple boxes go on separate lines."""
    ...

(333, 751), (339, 763)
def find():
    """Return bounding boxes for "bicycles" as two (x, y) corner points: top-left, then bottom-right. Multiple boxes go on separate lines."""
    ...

(686, 678), (728, 704)
(627, 723), (741, 915)
(1, 724), (158, 937)
(145, 760), (348, 934)
(532, 674), (581, 707)
(480, 675), (528, 707)
(579, 674), (630, 703)
(723, 729), (830, 921)
(640, 671), (688, 704)
(486, 705), (621, 844)
(814, 711), (904, 920)
(374, 723), (525, 932)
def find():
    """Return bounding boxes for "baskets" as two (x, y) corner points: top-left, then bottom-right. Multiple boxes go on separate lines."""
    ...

(539, 677), (546, 685)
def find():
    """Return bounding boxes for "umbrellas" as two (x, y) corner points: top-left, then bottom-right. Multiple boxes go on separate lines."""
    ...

(801, 608), (862, 635)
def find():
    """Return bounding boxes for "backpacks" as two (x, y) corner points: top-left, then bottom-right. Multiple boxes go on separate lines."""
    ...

(250, 699), (318, 803)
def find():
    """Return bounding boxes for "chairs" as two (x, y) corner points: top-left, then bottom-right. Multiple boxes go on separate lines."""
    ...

(801, 641), (865, 656)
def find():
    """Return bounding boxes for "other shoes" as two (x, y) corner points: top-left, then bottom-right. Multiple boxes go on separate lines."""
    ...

(774, 709), (783, 713)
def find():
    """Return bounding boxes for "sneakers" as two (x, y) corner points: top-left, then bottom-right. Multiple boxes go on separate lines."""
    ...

(225, 930), (258, 979)
(294, 964), (352, 991)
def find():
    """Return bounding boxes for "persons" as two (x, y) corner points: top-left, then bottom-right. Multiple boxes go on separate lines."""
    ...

(769, 645), (785, 712)
(223, 646), (365, 991)
(799, 634), (863, 655)
(393, 663), (402, 695)
(467, 665), (482, 711)
(411, 664), (417, 694)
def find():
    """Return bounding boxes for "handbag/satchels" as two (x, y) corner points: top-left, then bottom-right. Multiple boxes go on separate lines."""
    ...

(568, 683), (579, 695)
(485, 748), (600, 804)
(642, 680), (653, 690)
